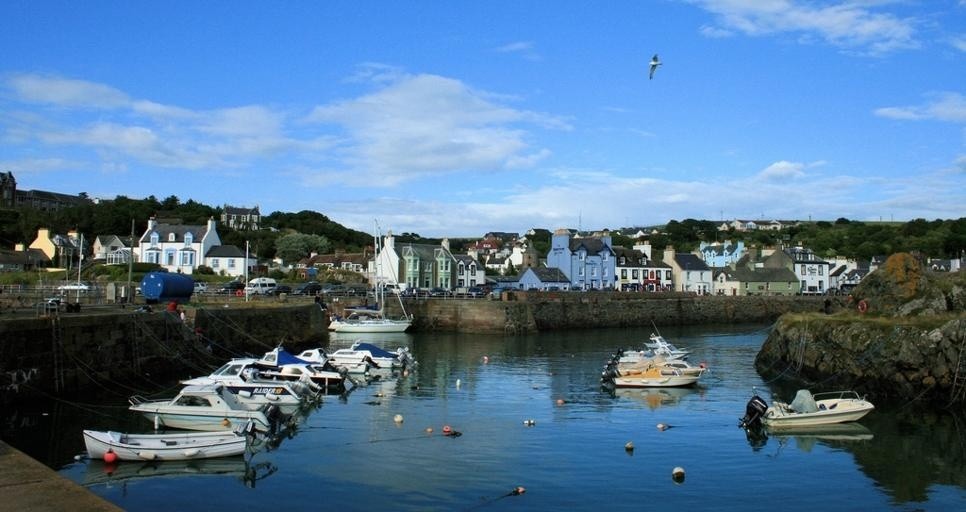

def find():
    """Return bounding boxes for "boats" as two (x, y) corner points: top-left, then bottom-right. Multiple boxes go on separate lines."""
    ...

(328, 306), (414, 331)
(83, 417), (257, 461)
(176, 340), (406, 417)
(83, 465), (275, 500)
(737, 389), (874, 427)
(746, 424), (872, 444)
(600, 333), (705, 394)
(127, 385), (287, 433)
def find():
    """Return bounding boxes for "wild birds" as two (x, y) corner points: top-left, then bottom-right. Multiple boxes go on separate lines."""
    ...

(649, 54), (663, 79)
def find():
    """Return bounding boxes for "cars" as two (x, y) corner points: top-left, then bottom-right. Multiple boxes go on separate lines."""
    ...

(34, 298), (81, 313)
(56, 280), (95, 291)
(215, 277), (507, 299)
(194, 282), (207, 294)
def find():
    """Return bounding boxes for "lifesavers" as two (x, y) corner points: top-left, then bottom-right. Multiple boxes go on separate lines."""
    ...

(858, 300), (866, 314)
(236, 290), (242, 296)
